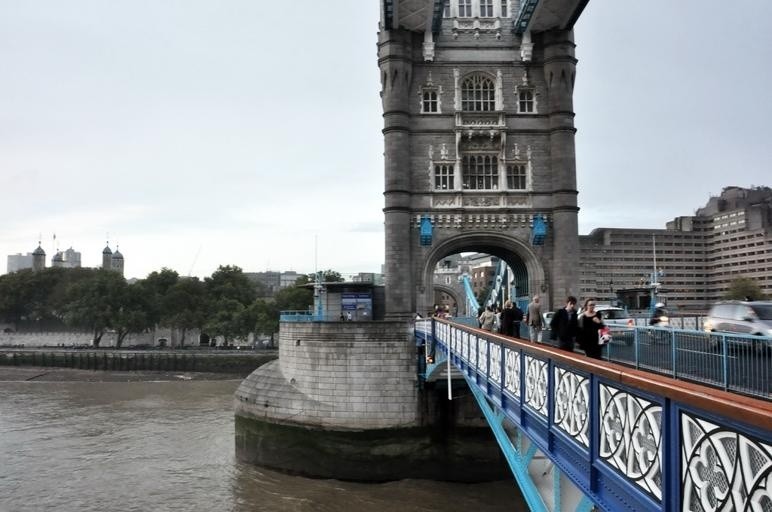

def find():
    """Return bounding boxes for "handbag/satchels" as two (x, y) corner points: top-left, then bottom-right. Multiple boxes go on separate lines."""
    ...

(491, 325), (498, 332)
(598, 327), (612, 344)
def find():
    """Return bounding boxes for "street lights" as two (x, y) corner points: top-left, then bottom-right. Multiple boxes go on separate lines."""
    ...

(313, 272), (323, 315)
(651, 270), (664, 311)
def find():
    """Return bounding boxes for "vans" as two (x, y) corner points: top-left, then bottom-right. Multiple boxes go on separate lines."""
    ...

(703, 296), (772, 356)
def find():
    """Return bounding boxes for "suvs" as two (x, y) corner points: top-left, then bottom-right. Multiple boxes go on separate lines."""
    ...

(576, 305), (635, 345)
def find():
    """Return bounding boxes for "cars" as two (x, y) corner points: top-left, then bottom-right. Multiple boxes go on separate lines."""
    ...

(541, 312), (557, 330)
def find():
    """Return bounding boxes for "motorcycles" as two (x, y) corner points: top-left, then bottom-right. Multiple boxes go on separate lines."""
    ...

(647, 316), (673, 346)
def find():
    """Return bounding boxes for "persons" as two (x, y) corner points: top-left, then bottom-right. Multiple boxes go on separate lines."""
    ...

(340, 312), (345, 321)
(478, 299), (523, 339)
(650, 302), (665, 324)
(523, 295), (545, 344)
(549, 297), (578, 352)
(347, 312), (352, 320)
(577, 298), (605, 360)
